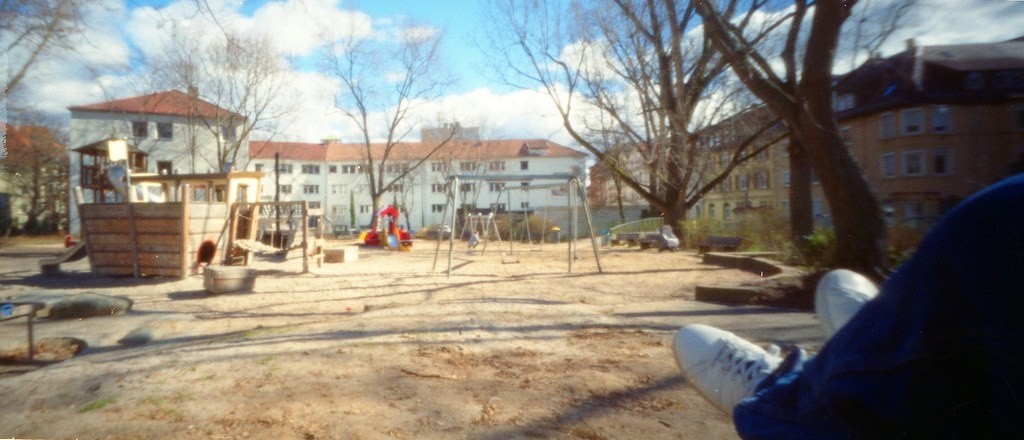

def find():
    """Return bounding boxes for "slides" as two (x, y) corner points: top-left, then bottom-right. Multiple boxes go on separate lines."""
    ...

(39, 240), (88, 267)
(384, 228), (409, 251)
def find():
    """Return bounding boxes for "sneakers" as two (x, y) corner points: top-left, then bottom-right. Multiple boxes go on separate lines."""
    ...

(672, 324), (784, 419)
(814, 269), (880, 339)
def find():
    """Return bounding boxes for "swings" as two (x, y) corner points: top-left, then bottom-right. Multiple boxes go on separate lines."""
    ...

(485, 179), (552, 265)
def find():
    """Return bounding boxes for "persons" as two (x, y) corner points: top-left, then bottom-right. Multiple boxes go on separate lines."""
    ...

(467, 231), (480, 249)
(670, 172), (1024, 440)
